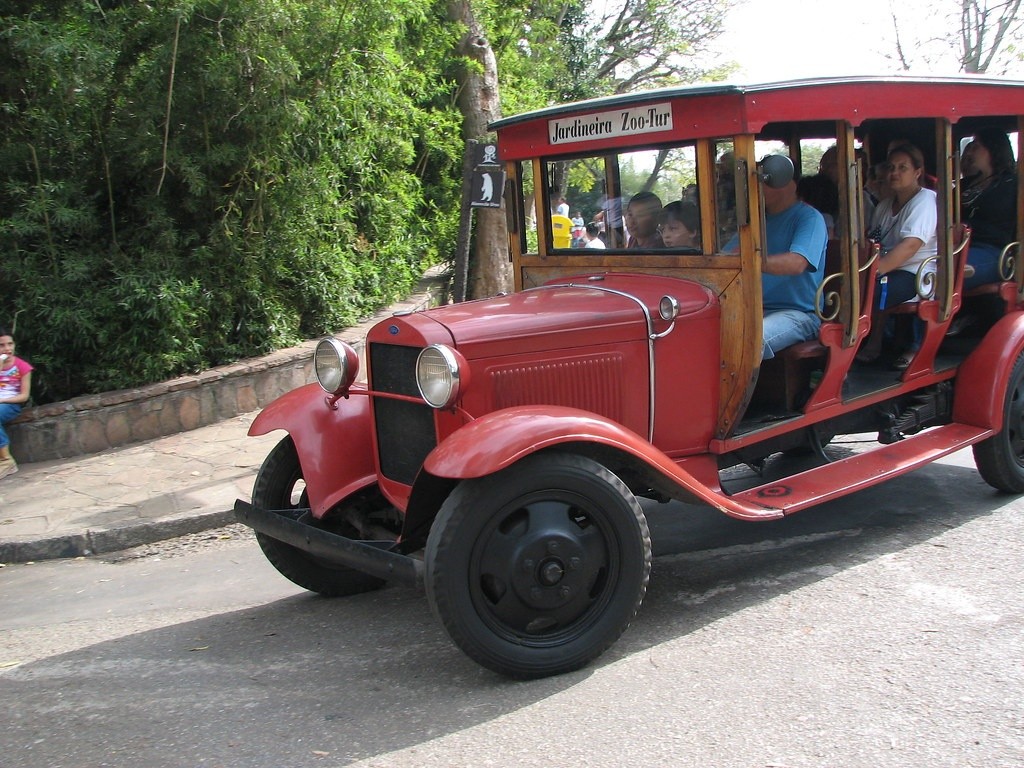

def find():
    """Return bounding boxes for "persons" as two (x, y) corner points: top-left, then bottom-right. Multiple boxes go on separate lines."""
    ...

(683, 127), (1017, 369)
(0, 332), (34, 480)
(658, 201), (700, 249)
(557, 197), (607, 249)
(624, 192), (665, 248)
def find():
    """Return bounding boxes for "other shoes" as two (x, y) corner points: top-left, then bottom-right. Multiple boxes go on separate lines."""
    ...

(1, 457), (19, 480)
(893, 352), (916, 370)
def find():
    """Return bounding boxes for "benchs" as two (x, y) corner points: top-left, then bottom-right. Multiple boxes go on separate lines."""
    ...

(965, 240), (1020, 313)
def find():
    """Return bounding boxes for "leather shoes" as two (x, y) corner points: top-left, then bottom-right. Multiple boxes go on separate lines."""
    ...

(946, 313), (978, 337)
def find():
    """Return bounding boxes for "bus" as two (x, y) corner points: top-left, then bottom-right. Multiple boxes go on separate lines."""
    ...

(230, 78), (1024, 679)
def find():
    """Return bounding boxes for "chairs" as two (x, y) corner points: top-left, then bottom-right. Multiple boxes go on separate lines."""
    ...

(760, 237), (881, 415)
(872, 221), (972, 388)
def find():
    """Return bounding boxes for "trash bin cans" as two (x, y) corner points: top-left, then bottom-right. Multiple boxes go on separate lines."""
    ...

(418, 261), (456, 308)
(551, 214), (575, 249)
